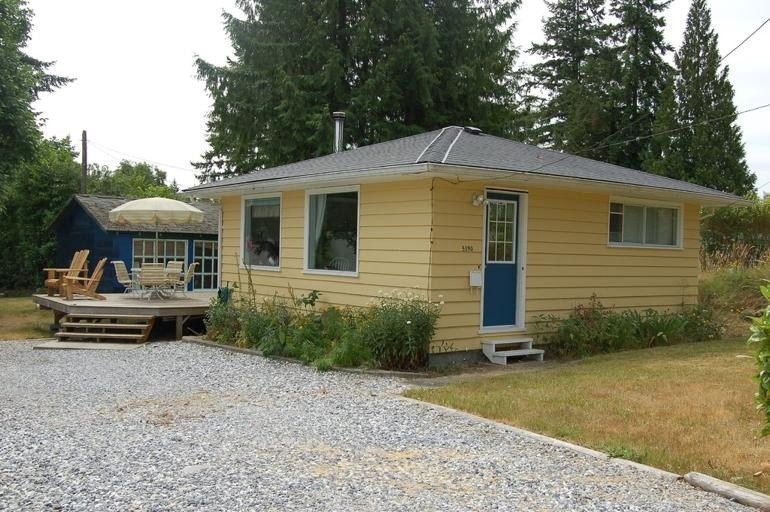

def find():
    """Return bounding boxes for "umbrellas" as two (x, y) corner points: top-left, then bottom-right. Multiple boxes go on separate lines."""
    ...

(109, 196), (207, 263)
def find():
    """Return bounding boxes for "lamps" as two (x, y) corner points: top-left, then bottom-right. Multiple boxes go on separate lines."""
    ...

(472, 193), (489, 207)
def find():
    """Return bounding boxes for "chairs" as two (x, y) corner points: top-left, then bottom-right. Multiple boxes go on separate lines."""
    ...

(44, 250), (200, 304)
